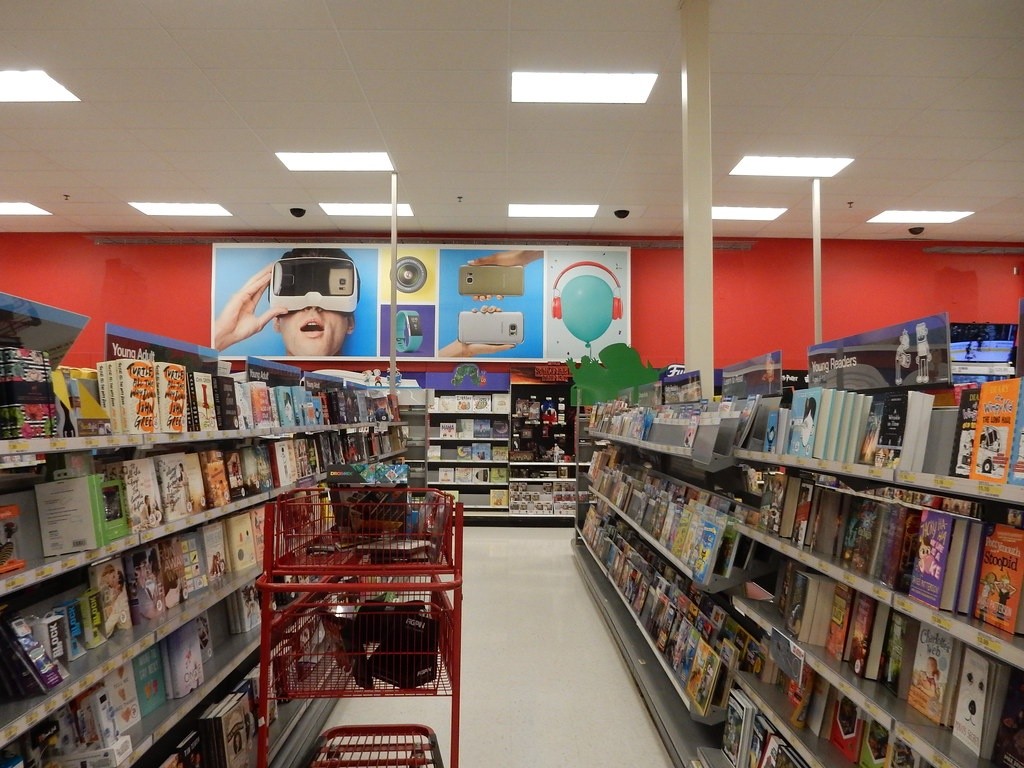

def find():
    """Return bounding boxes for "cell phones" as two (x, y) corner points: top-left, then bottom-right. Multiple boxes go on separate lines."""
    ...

(459, 265), (526, 296)
(458, 312), (524, 345)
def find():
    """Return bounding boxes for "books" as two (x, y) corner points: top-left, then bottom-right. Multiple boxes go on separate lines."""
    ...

(429, 383), (1024, 768)
(0, 358), (416, 768)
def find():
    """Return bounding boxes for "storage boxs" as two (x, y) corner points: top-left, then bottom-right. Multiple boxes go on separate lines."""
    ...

(324, 461), (412, 541)
(0, 473), (135, 768)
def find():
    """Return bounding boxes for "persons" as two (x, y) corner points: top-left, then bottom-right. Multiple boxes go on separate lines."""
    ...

(213, 248), (362, 356)
(139, 496), (152, 522)
(174, 461), (192, 512)
(104, 573), (131, 636)
(132, 551), (157, 619)
(234, 734), (242, 753)
(98, 565), (117, 612)
(439, 249), (544, 357)
(242, 586), (262, 628)
(147, 548), (166, 616)
(195, 617), (210, 648)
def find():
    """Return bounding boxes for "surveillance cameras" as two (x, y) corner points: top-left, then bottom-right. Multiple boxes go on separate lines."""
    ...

(614, 210), (629, 218)
(909, 227), (924, 235)
(290, 208), (305, 218)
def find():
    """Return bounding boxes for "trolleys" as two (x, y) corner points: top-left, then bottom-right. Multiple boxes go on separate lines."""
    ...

(256, 484), (466, 768)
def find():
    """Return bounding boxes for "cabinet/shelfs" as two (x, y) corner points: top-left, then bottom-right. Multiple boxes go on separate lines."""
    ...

(0, 421), (409, 768)
(398, 387), (576, 528)
(569, 427), (1024, 768)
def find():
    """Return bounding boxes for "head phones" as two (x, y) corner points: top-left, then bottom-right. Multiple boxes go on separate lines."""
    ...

(552, 262), (623, 320)
